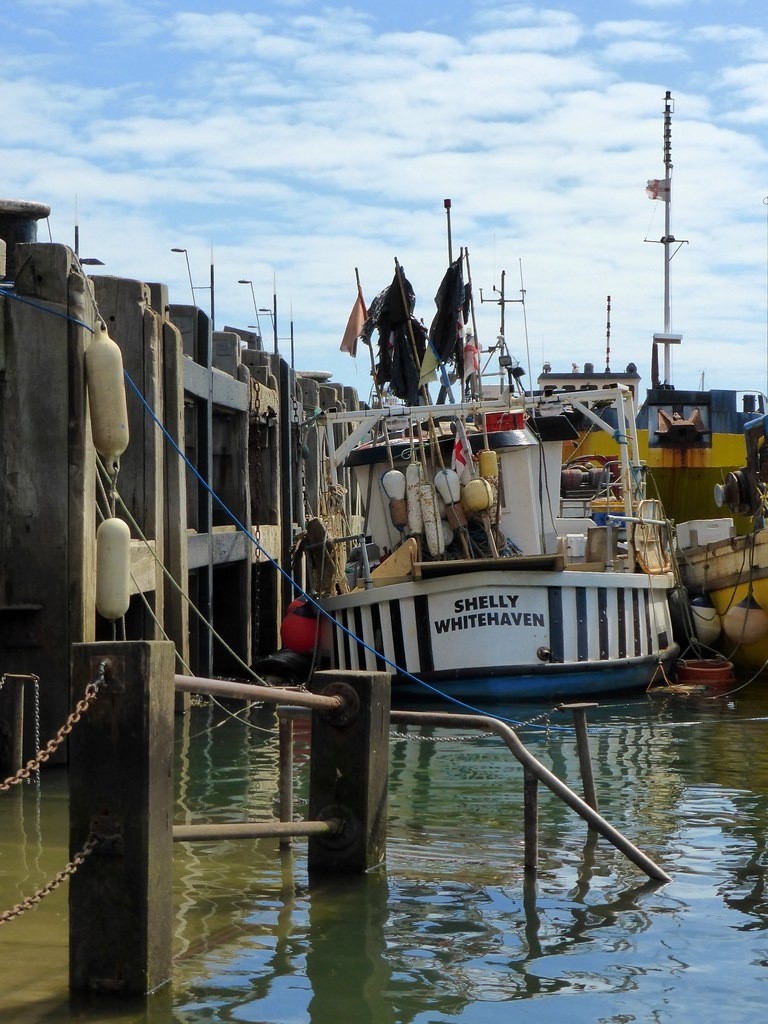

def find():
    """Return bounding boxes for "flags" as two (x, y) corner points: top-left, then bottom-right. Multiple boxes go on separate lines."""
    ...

(340, 257), (478, 404)
(452, 425), (475, 486)
(647, 180), (669, 202)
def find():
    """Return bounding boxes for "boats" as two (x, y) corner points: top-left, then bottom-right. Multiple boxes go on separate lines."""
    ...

(313, 382), (681, 700)
(537, 89), (768, 669)
